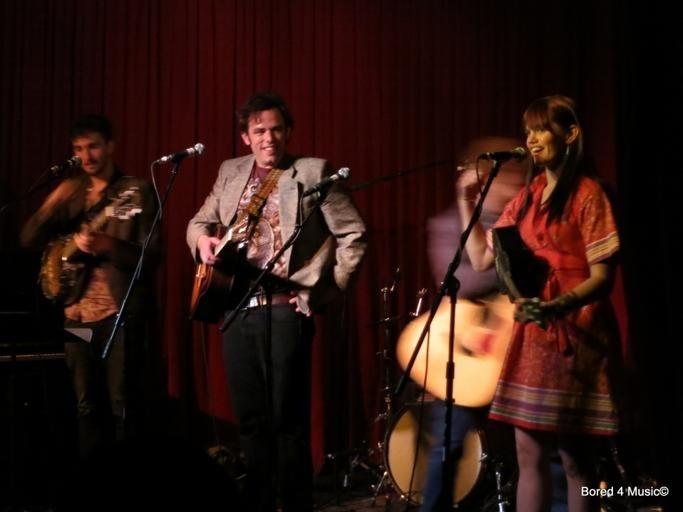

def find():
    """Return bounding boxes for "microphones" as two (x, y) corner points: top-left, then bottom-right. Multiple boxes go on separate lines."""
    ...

(478, 147), (528, 162)
(155, 142), (205, 166)
(303, 167), (351, 198)
(51, 156), (81, 175)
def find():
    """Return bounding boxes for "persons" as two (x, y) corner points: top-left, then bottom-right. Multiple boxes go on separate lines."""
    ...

(420, 96), (630, 512)
(18, 113), (162, 462)
(185, 90), (367, 511)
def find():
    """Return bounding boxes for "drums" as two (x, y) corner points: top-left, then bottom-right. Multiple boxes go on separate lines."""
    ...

(382, 408), (505, 507)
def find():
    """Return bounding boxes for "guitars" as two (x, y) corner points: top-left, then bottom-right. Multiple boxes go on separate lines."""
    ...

(492, 224), (550, 332)
(191, 214), (253, 320)
(396, 284), (515, 408)
(39, 187), (138, 301)
